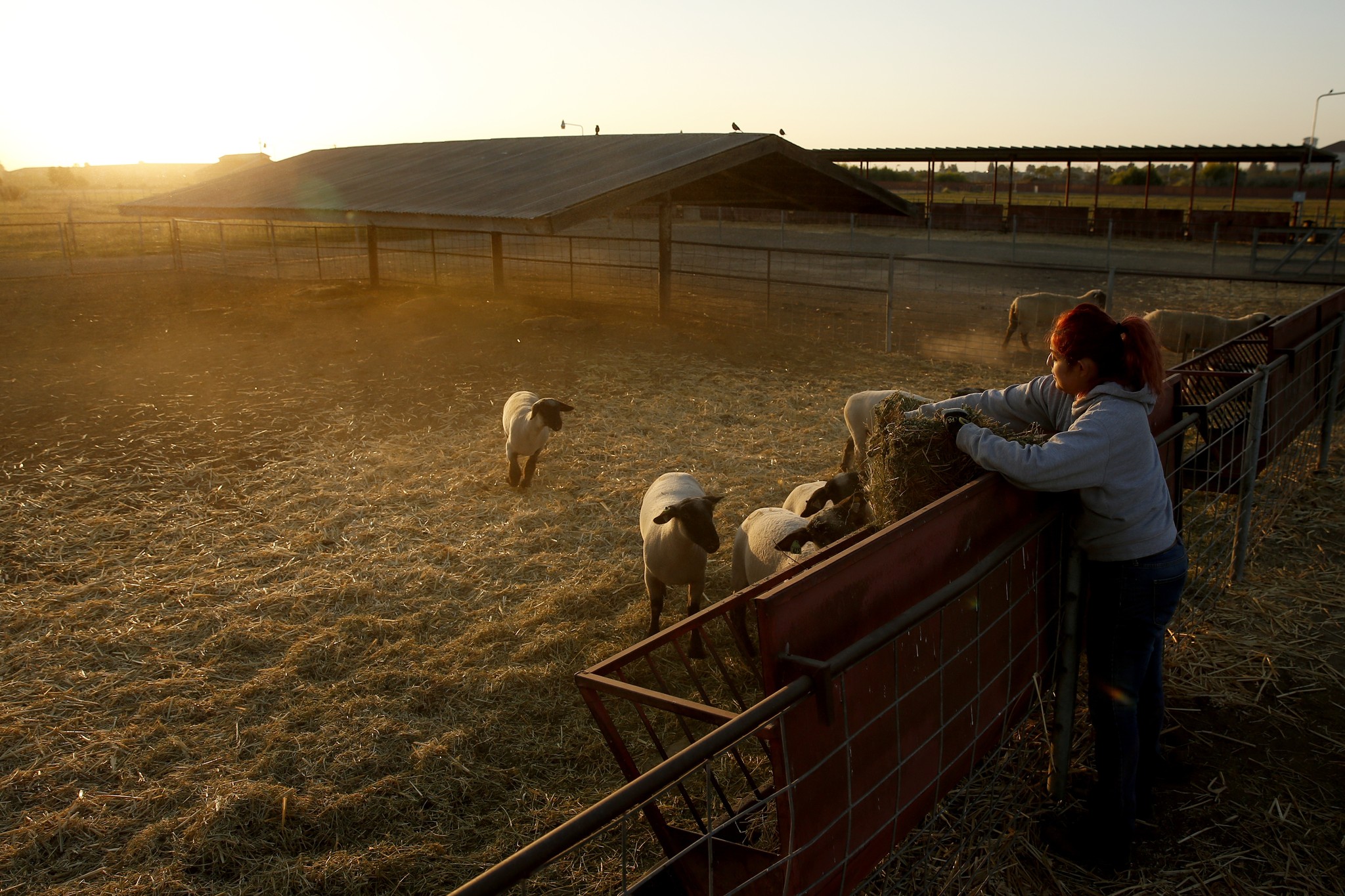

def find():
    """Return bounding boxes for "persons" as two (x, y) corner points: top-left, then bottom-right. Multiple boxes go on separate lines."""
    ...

(902, 303), (1189, 882)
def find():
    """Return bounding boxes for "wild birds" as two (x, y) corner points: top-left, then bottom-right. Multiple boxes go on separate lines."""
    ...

(780, 129), (787, 135)
(595, 125), (599, 135)
(732, 122), (744, 133)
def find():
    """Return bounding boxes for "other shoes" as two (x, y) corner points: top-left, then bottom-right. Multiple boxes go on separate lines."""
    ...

(1038, 768), (1155, 868)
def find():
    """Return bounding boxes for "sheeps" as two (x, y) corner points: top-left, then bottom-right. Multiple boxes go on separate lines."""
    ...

(1143, 308), (1271, 354)
(1002, 288), (1107, 352)
(501, 390), (575, 493)
(639, 471), (726, 660)
(727, 387), (987, 658)
